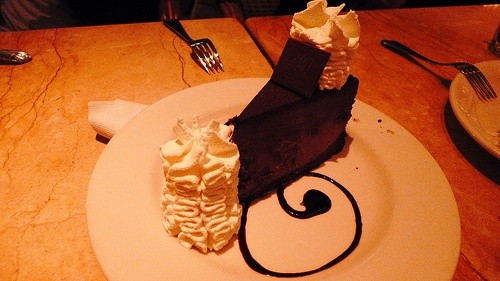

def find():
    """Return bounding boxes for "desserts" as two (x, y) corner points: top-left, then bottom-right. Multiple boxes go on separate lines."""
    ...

(157, 0), (360, 252)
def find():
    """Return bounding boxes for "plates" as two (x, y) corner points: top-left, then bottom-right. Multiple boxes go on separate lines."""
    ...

(449, 61), (500, 160)
(85, 75), (460, 281)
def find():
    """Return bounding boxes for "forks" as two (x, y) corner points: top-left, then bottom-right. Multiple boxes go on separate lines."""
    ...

(163, 17), (225, 76)
(380, 39), (498, 102)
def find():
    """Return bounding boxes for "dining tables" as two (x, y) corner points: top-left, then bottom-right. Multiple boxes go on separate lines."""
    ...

(0, 4), (500, 281)
